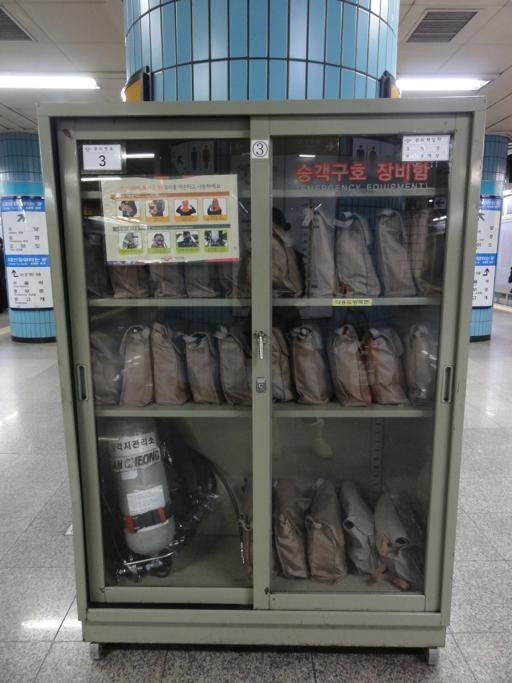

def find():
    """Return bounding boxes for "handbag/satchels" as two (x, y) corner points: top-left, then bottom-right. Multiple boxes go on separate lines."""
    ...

(107, 227), (250, 298)
(273, 476), (425, 587)
(272, 323), (436, 405)
(88, 324), (248, 408)
(271, 210), (437, 298)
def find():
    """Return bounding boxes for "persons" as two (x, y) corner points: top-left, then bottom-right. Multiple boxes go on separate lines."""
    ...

(121, 232), (138, 247)
(150, 233), (167, 248)
(176, 230), (198, 247)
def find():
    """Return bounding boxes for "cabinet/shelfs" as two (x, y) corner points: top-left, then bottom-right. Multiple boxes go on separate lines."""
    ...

(33, 95), (487, 670)
